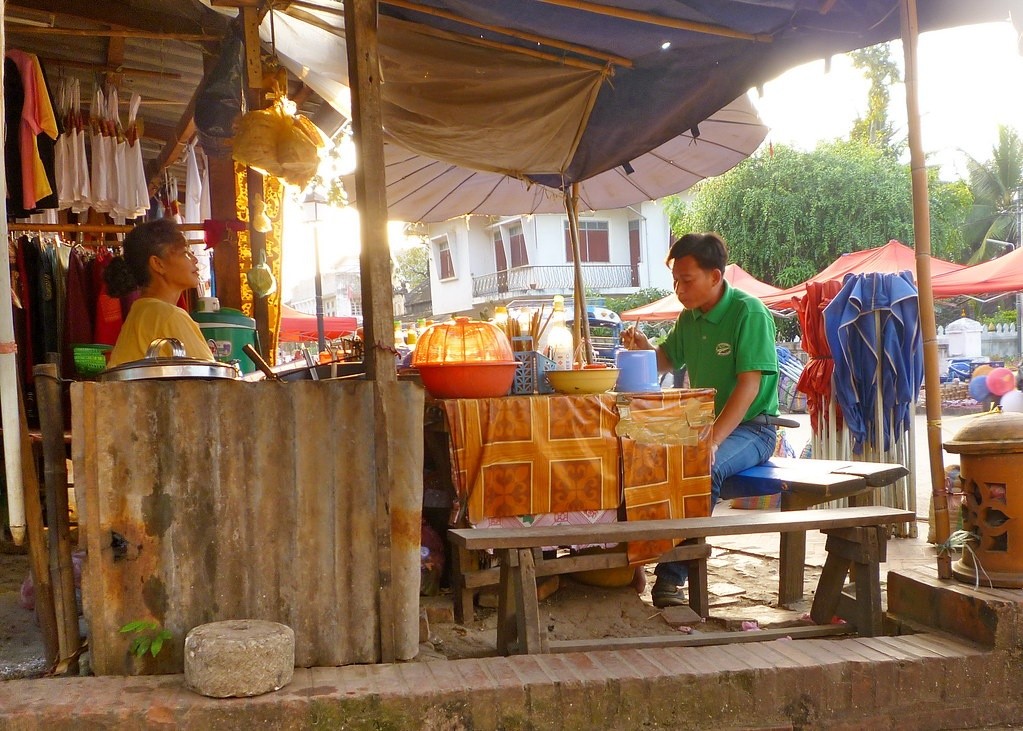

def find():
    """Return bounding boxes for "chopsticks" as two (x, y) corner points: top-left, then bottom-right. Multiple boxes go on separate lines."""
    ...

(496, 304), (555, 353)
(628, 317), (640, 350)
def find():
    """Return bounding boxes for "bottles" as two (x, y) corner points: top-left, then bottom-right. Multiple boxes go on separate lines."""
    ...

(547, 295), (573, 368)
(394, 318), (434, 345)
(495, 307), (507, 323)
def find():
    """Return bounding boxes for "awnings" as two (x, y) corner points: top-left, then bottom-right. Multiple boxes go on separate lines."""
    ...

(4, 0), (1018, 221)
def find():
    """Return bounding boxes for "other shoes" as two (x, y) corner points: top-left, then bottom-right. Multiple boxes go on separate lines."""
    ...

(652, 579), (684, 607)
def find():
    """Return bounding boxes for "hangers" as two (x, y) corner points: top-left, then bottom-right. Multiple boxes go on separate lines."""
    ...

(53, 59), (139, 101)
(9, 222), (125, 253)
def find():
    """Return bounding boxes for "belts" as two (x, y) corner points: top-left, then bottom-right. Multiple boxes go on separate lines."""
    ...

(750, 412), (800, 428)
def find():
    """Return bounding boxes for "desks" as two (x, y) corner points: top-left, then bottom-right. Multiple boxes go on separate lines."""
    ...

(425, 387), (717, 624)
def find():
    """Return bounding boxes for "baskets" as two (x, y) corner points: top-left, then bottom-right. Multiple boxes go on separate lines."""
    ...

(513, 351), (557, 394)
(74, 342), (113, 378)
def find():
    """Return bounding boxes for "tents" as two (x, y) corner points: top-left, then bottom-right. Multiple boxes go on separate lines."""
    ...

(277, 302), (358, 343)
(792, 271), (925, 540)
(620, 240), (1022, 320)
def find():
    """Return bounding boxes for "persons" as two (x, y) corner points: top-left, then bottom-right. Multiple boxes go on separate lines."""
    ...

(619, 234), (779, 605)
(104, 218), (217, 369)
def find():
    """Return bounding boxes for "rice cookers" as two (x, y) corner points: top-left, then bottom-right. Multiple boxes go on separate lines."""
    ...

(191, 307), (257, 374)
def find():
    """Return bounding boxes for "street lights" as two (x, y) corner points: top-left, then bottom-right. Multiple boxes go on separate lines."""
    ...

(301, 188), (328, 354)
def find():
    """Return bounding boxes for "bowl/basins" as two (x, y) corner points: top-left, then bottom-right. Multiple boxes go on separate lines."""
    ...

(414, 361), (523, 397)
(542, 368), (622, 393)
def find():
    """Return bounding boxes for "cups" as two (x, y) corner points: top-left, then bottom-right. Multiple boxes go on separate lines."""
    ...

(197, 297), (220, 314)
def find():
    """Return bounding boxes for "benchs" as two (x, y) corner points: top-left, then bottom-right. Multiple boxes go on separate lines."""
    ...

(448, 506), (915, 655)
(719, 458), (909, 605)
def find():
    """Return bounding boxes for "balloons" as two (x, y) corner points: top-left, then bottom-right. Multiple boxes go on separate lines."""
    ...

(969, 365), (1023, 413)
